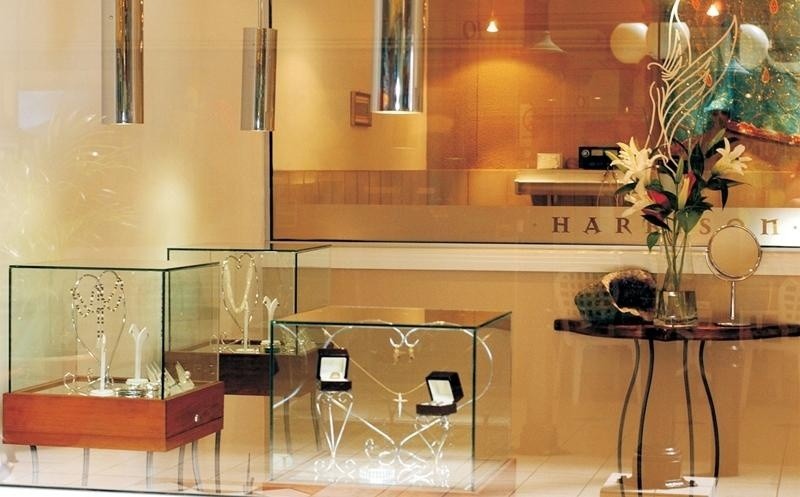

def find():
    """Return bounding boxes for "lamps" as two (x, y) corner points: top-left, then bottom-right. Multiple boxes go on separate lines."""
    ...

(485, 0), (499, 32)
(645, 6), (691, 62)
(529, 1), (564, 53)
(719, 21), (769, 71)
(610, 20), (648, 64)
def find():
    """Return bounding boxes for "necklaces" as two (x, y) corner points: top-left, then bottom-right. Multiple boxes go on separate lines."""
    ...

(65, 276), (124, 340)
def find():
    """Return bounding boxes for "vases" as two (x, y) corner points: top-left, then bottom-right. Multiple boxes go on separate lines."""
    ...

(656, 236), (699, 327)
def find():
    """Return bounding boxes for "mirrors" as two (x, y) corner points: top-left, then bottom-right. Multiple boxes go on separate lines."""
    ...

(706, 224), (762, 326)
(257, 1), (800, 253)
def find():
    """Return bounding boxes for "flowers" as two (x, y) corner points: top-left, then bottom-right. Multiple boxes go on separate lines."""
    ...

(594, 131), (751, 290)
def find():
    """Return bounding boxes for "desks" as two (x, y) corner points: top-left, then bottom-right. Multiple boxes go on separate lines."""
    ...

(515, 167), (624, 206)
(554, 316), (799, 497)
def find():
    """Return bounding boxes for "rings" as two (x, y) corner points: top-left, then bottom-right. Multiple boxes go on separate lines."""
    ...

(329, 372), (341, 378)
(429, 400), (444, 406)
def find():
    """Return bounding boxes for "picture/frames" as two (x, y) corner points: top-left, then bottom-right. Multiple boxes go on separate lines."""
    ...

(350, 90), (373, 127)
